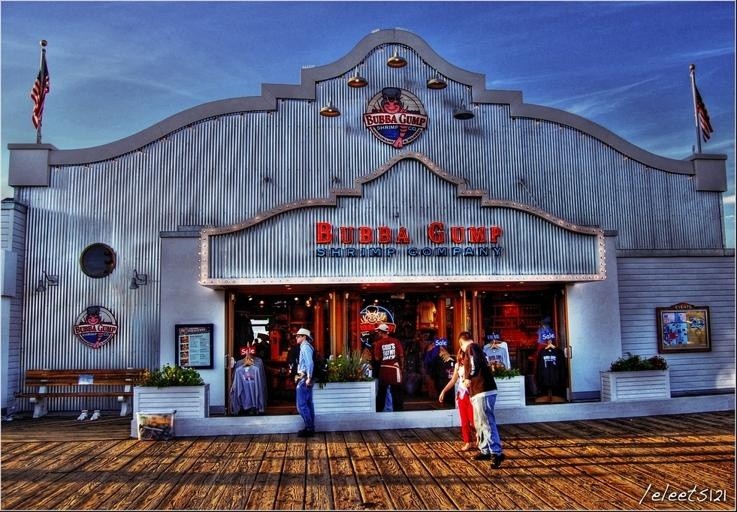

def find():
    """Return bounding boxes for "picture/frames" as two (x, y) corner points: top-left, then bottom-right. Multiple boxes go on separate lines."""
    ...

(174, 322), (215, 370)
(655, 303), (713, 353)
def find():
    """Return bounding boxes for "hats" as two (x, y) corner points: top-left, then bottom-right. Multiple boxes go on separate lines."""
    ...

(376, 323), (389, 332)
(292, 326), (314, 342)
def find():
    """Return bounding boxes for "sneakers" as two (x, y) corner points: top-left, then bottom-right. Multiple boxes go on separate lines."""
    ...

(90, 407), (101, 420)
(489, 452), (505, 469)
(297, 427), (315, 438)
(459, 442), (472, 452)
(470, 452), (491, 461)
(76, 406), (88, 421)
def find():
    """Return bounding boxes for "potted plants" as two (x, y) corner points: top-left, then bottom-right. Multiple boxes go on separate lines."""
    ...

(491, 369), (525, 408)
(599, 351), (671, 401)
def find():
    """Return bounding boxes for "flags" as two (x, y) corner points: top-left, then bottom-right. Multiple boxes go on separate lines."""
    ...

(693, 84), (713, 142)
(28, 55), (52, 130)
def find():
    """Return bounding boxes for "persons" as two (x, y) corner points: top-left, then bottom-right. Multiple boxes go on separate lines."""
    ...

(292, 327), (316, 438)
(437, 348), (478, 452)
(370, 324), (405, 412)
(458, 330), (505, 468)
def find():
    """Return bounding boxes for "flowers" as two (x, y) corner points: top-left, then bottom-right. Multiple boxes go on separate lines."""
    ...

(135, 364), (204, 387)
(315, 343), (373, 387)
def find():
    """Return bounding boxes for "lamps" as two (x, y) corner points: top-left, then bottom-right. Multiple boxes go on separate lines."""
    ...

(347, 72), (368, 88)
(129, 269), (147, 290)
(426, 74), (447, 90)
(453, 97), (475, 119)
(319, 101), (340, 117)
(387, 50), (407, 68)
(35, 271), (58, 293)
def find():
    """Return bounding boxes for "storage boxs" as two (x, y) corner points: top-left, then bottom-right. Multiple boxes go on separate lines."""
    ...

(135, 410), (177, 441)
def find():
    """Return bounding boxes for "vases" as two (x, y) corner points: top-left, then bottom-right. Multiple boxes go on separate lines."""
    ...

(133, 384), (211, 418)
(313, 379), (378, 414)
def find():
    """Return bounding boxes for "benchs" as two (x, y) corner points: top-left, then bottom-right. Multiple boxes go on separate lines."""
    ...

(13, 368), (147, 419)
